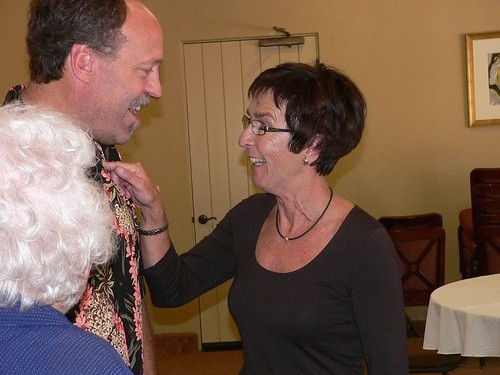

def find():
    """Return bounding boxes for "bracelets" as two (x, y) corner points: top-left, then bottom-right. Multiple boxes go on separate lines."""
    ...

(139, 222), (169, 236)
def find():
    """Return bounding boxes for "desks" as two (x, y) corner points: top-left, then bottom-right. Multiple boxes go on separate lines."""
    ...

(423, 273), (500, 357)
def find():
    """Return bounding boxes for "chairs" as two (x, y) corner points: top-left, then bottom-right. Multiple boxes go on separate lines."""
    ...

(378, 168), (500, 375)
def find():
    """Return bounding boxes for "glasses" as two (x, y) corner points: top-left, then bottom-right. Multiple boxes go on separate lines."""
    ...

(241, 113), (296, 137)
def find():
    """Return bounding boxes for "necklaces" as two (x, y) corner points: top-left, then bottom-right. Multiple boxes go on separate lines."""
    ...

(84, 140), (124, 170)
(276, 187), (333, 242)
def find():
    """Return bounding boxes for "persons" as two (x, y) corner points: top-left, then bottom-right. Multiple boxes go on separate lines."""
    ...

(0, 0), (165, 375)
(0, 102), (134, 375)
(101, 62), (410, 375)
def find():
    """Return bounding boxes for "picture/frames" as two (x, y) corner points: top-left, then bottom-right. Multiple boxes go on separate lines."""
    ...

(464, 31), (500, 127)
